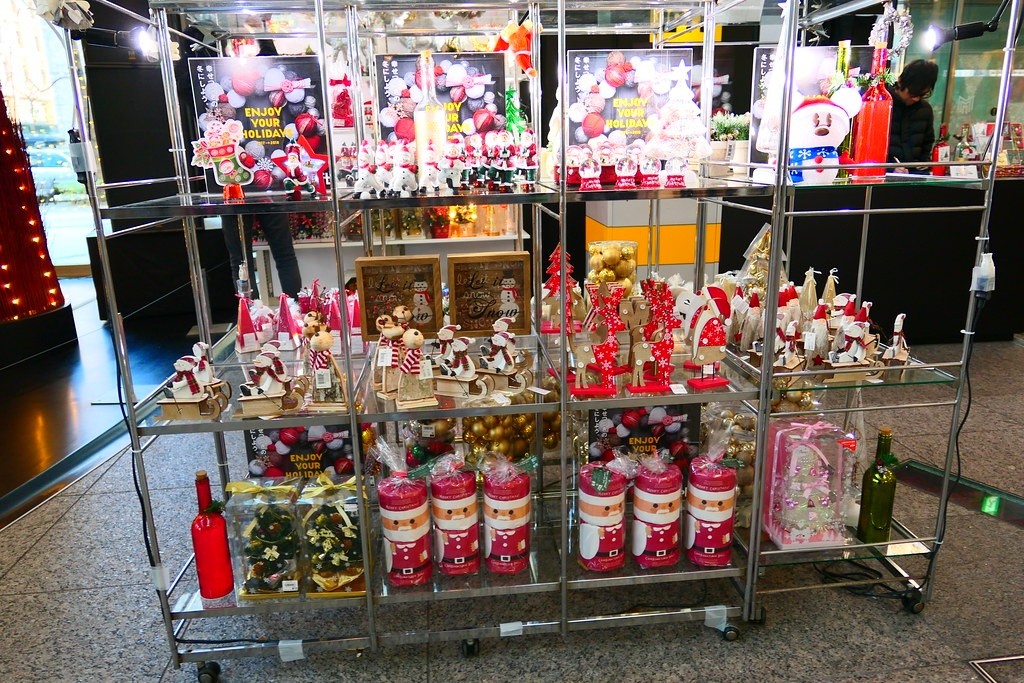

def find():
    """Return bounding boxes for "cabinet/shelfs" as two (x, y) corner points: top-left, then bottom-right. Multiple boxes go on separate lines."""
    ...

(65, 0), (1024, 683)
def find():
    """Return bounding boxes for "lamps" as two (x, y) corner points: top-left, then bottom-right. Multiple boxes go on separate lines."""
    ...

(925, 0), (1010, 52)
(70, 23), (148, 59)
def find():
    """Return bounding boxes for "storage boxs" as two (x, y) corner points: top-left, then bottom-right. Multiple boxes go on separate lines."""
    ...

(761, 416), (849, 551)
(446, 251), (531, 338)
(356, 251), (444, 341)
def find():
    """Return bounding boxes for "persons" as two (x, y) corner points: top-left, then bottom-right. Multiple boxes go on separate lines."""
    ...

(221, 215), (303, 302)
(885, 58), (939, 176)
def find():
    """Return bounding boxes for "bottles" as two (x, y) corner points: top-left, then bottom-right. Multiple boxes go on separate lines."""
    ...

(412, 49), (447, 184)
(931, 122), (970, 176)
(828, 38), (890, 184)
(190, 470), (234, 600)
(856, 425), (898, 545)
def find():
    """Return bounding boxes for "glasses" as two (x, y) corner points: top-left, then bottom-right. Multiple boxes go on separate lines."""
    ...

(907, 87), (933, 99)
